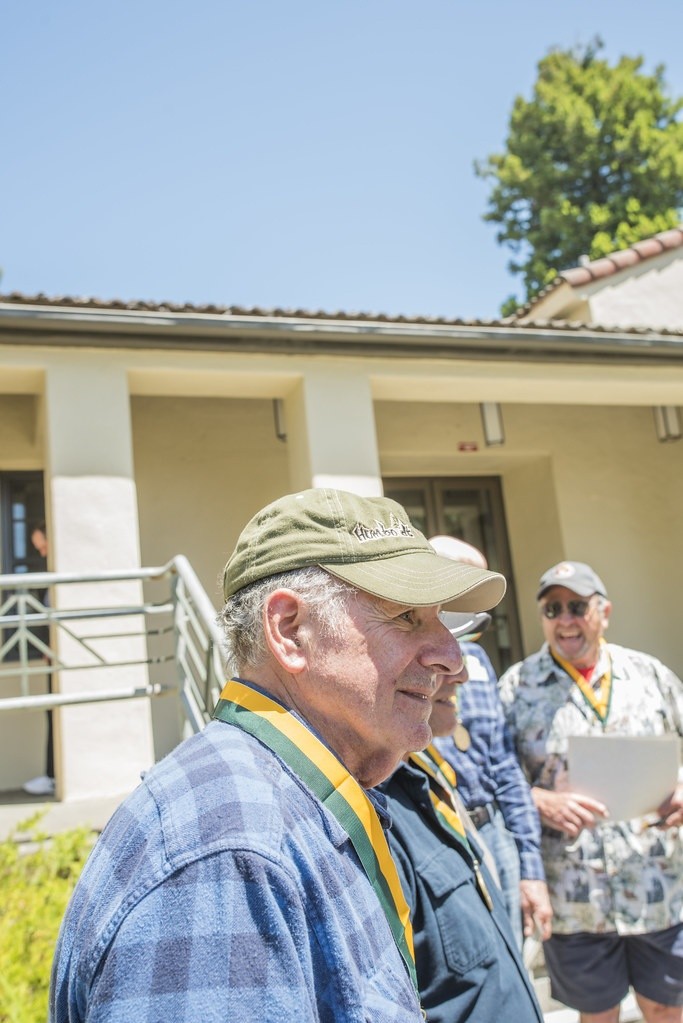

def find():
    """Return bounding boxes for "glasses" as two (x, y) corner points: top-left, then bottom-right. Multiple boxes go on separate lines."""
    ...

(539, 601), (589, 619)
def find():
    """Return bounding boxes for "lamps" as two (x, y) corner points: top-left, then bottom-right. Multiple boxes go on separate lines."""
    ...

(274, 397), (288, 443)
(652, 404), (683, 444)
(479, 401), (509, 448)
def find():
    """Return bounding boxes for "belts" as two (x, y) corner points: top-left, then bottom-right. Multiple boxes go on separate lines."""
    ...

(470, 801), (497, 830)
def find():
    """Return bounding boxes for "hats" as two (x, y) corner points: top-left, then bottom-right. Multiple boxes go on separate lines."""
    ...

(535, 561), (607, 601)
(221, 488), (507, 613)
(427, 534), (492, 640)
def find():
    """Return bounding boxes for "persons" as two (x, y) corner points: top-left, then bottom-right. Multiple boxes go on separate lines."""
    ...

(495, 560), (682, 1023)
(22, 522), (56, 795)
(367, 665), (545, 1022)
(49, 488), (507, 1023)
(426, 534), (552, 958)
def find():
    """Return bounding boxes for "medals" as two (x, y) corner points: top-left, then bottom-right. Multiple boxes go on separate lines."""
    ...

(452, 726), (470, 752)
(476, 871), (493, 912)
